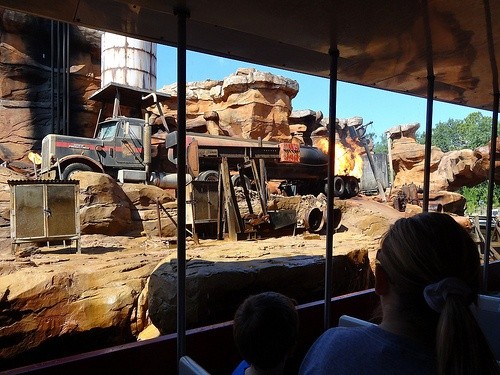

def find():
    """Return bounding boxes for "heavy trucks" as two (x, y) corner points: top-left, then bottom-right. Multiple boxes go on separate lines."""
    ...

(31, 92), (363, 200)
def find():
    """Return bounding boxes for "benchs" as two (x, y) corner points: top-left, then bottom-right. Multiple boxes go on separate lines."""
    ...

(478, 293), (500, 359)
(179, 355), (210, 375)
(337, 314), (375, 326)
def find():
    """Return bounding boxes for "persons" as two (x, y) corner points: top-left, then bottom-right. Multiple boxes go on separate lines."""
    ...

(231, 289), (301, 375)
(291, 205), (497, 375)
